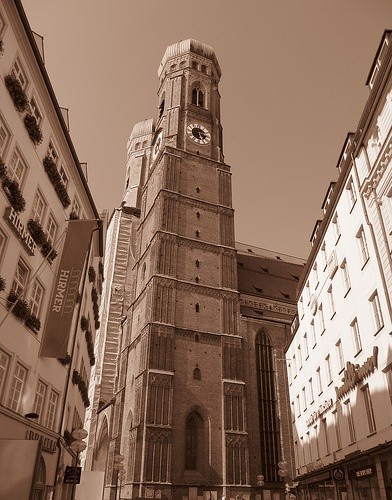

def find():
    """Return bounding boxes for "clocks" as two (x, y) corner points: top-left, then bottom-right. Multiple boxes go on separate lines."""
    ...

(187, 123), (212, 146)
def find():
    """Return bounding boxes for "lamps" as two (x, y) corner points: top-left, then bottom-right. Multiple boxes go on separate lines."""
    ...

(25, 413), (39, 420)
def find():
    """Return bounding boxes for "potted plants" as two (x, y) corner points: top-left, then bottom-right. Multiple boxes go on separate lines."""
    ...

(76, 292), (98, 367)
(0, 156), (26, 215)
(28, 219), (58, 260)
(0, 275), (41, 331)
(59, 352), (91, 407)
(24, 114), (44, 145)
(43, 155), (71, 210)
(5, 73), (29, 113)
(89, 261), (105, 330)
